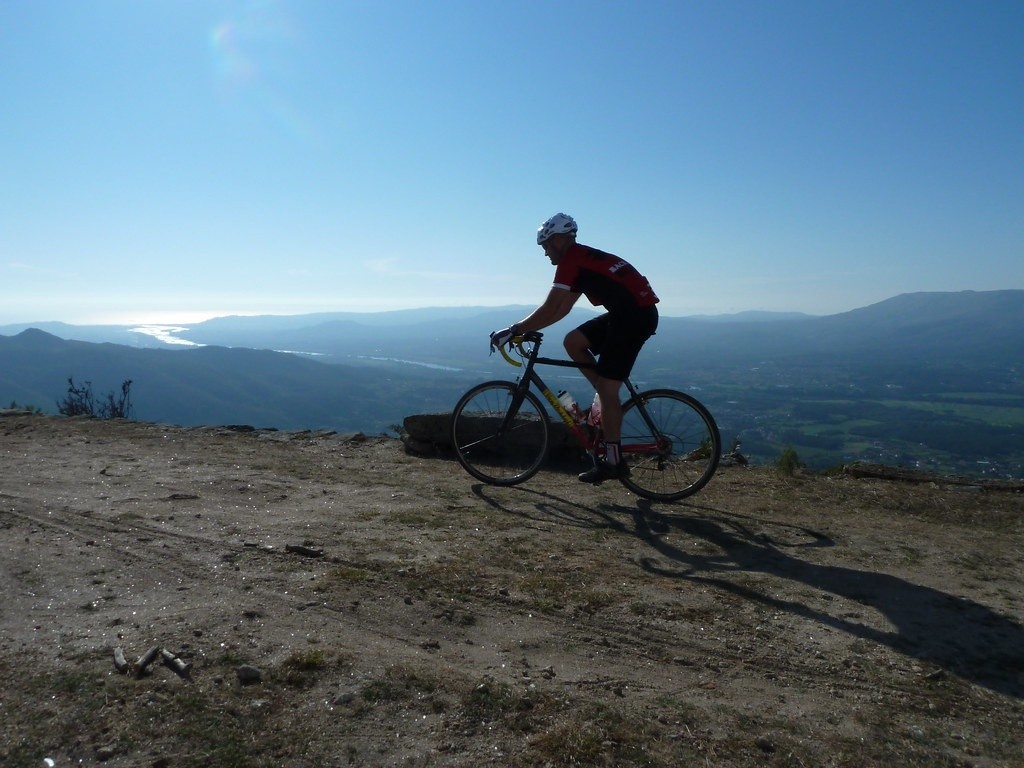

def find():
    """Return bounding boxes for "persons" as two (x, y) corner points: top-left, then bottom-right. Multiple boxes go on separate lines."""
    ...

(492, 213), (661, 483)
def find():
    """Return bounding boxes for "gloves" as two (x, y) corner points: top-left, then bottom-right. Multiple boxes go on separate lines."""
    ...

(490, 327), (514, 348)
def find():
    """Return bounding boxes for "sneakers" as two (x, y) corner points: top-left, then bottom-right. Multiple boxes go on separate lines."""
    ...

(578, 458), (631, 486)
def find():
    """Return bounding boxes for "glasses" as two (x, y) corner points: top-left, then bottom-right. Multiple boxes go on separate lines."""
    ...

(542, 236), (557, 249)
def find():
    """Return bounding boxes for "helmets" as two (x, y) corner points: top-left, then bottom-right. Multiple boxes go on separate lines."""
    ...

(537, 212), (578, 245)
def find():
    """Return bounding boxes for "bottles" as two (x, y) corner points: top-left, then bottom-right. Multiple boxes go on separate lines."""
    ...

(558, 389), (586, 424)
(588, 393), (601, 429)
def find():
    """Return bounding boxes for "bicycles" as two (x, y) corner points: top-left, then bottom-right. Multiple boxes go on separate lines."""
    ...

(449, 331), (721, 502)
